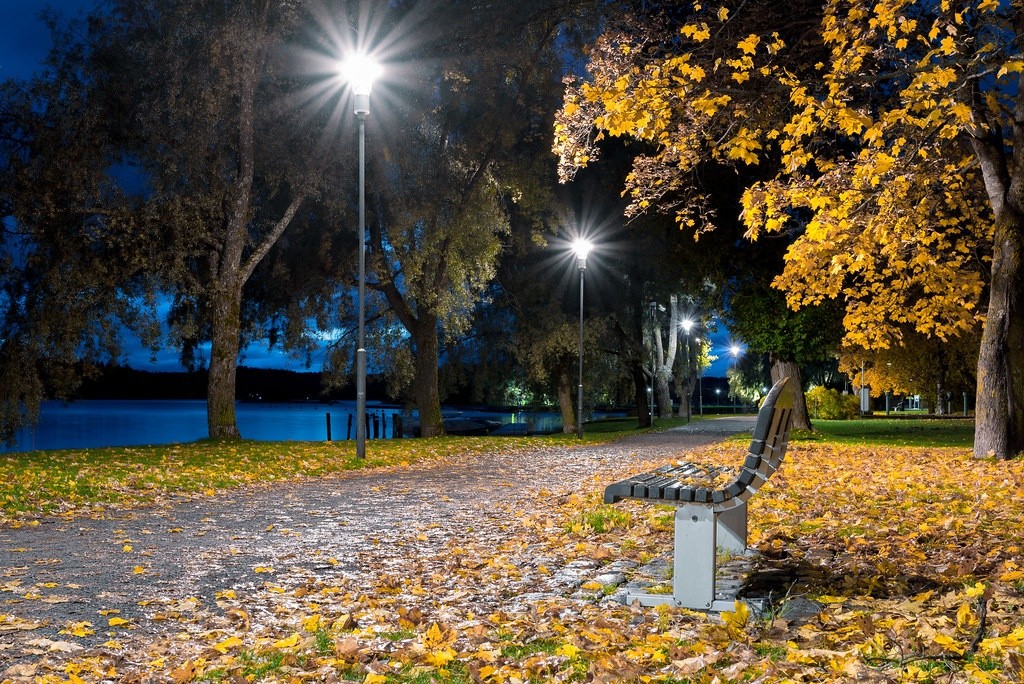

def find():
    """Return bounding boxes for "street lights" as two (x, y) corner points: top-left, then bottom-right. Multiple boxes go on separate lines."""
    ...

(569, 234), (593, 440)
(681, 319), (694, 424)
(333, 48), (381, 459)
(731, 343), (738, 414)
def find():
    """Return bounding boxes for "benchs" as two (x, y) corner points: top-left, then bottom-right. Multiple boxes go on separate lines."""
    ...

(892, 406), (902, 411)
(603, 375), (794, 614)
